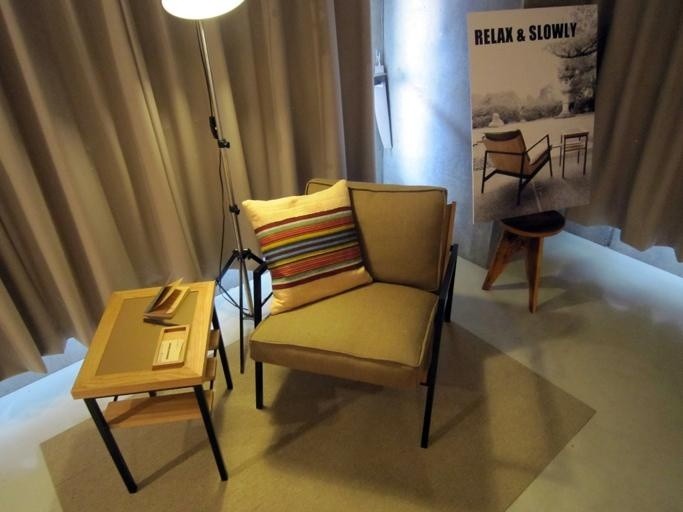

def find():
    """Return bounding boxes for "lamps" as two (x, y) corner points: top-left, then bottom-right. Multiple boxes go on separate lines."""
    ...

(160, 1), (267, 375)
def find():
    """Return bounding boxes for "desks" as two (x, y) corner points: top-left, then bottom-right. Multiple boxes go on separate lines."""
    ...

(70, 280), (233, 494)
(559, 128), (588, 177)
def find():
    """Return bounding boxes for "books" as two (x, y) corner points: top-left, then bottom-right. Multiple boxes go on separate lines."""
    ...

(143, 271), (190, 319)
(159, 291), (199, 328)
(151, 326), (191, 369)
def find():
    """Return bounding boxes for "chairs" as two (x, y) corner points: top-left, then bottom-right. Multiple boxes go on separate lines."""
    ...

(249, 176), (459, 448)
(481, 129), (553, 208)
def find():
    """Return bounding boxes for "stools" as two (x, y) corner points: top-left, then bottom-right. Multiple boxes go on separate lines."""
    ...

(482, 210), (567, 314)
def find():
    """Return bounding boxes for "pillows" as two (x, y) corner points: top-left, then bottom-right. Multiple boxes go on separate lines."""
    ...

(242, 179), (374, 316)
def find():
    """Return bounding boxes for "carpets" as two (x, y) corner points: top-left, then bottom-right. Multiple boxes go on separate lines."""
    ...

(40, 316), (595, 512)
